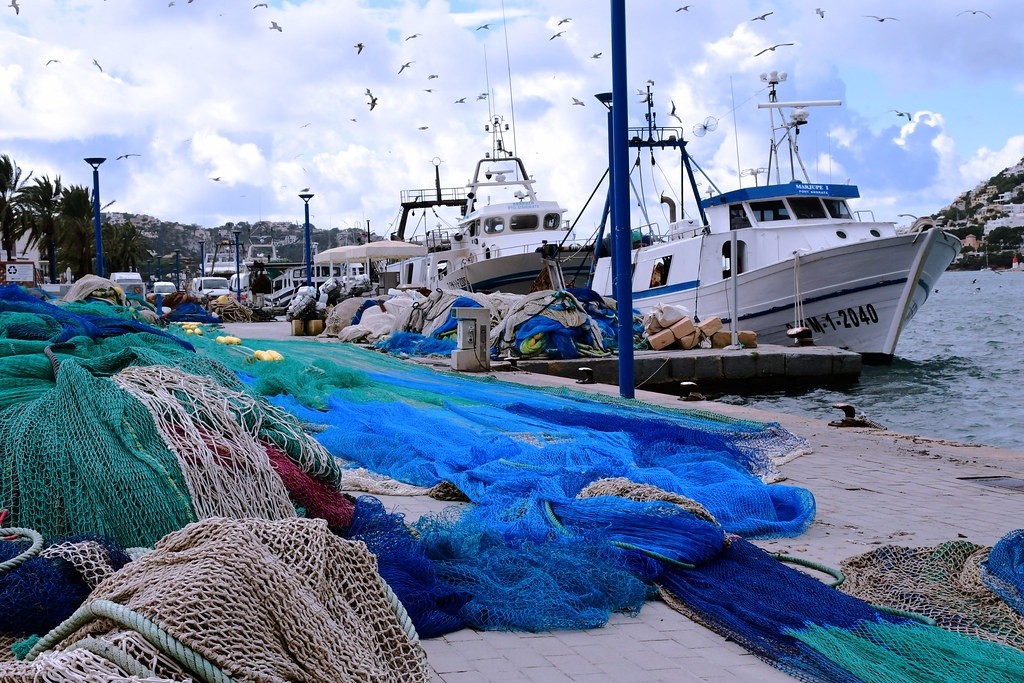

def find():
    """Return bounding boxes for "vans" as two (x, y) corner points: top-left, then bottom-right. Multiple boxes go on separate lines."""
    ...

(150, 282), (177, 297)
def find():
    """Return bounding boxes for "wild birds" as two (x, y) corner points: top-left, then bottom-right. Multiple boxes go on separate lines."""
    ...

(752, 12), (774, 22)
(675, 5), (694, 12)
(6, 0), (20, 15)
(636, 79), (654, 103)
(934, 278), (1003, 293)
(168, 0), (194, 6)
(351, 34), (490, 130)
(253, 3), (282, 32)
(550, 18), (572, 40)
(591, 52), (601, 58)
(864, 15), (900, 22)
(815, 8), (826, 18)
(957, 10), (991, 19)
(209, 177), (222, 181)
(476, 24), (491, 30)
(888, 110), (911, 121)
(116, 154), (141, 160)
(668, 100), (682, 123)
(46, 60), (61, 66)
(295, 123), (310, 172)
(93, 59), (102, 72)
(755, 43), (795, 58)
(572, 97), (585, 107)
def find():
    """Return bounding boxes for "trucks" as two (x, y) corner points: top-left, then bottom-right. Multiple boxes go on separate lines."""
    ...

(190, 276), (230, 299)
(109, 272), (142, 284)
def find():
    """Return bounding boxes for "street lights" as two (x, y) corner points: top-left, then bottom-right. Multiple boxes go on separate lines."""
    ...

(173, 249), (181, 291)
(156, 255), (162, 281)
(593, 91), (617, 301)
(146, 260), (153, 290)
(84, 157), (108, 277)
(231, 229), (243, 304)
(197, 241), (206, 277)
(297, 193), (315, 287)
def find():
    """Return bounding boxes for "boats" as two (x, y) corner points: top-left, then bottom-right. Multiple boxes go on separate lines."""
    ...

(200, 0), (598, 296)
(587, 76), (963, 365)
